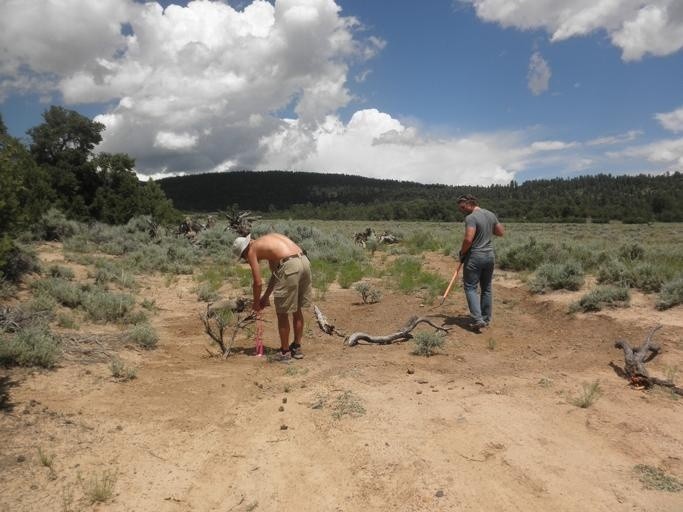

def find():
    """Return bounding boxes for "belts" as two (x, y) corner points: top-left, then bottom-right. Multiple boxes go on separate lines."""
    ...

(278, 252), (306, 266)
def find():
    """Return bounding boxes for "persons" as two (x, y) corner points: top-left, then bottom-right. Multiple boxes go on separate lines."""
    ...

(455, 192), (504, 332)
(232, 231), (315, 361)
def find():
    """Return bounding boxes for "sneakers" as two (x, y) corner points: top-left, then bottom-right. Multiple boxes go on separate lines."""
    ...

(288, 342), (304, 359)
(465, 316), (491, 331)
(270, 350), (291, 362)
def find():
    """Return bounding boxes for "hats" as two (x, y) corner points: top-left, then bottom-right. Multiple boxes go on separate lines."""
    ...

(231, 234), (250, 262)
